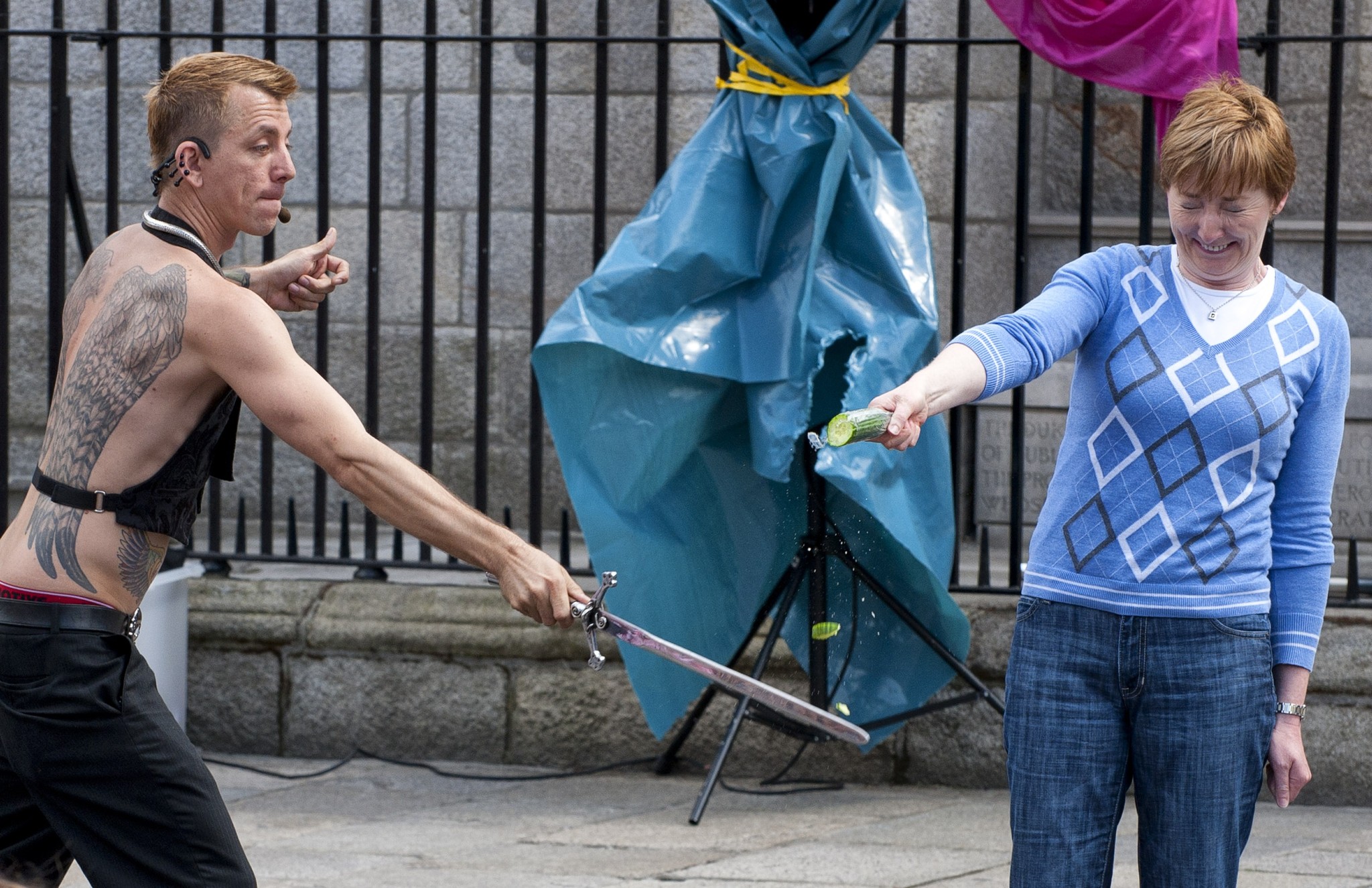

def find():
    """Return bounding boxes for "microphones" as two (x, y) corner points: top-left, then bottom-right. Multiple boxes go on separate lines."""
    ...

(279, 206), (291, 223)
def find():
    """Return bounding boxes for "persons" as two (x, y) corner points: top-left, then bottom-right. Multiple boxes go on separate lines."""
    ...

(867, 74), (1350, 888)
(0, 56), (594, 888)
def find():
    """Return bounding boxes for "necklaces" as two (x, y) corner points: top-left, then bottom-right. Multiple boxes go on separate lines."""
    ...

(1176, 260), (1261, 321)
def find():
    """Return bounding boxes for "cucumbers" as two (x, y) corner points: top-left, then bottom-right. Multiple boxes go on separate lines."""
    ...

(828, 408), (893, 446)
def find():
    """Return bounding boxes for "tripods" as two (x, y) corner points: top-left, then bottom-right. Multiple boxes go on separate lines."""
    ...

(657, 421), (1007, 827)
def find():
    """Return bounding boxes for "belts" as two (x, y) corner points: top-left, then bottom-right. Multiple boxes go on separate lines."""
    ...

(0, 598), (142, 641)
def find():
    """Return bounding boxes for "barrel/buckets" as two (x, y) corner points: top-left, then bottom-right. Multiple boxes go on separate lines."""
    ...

(132, 559), (206, 733)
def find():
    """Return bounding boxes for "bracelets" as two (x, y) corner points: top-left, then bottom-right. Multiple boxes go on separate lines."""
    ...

(1276, 702), (1307, 719)
